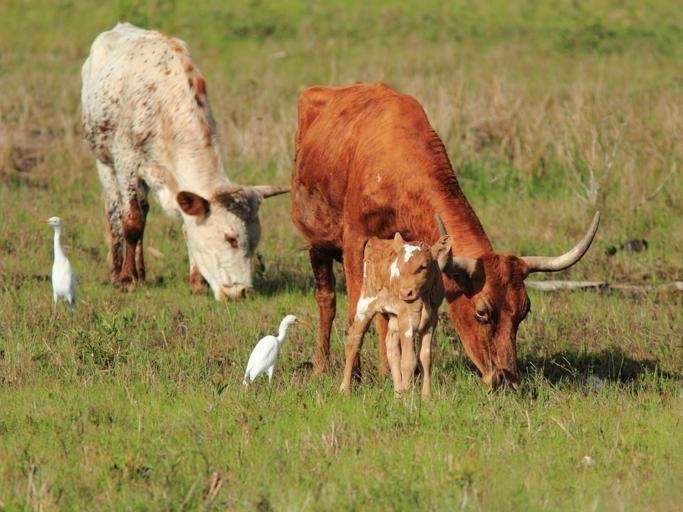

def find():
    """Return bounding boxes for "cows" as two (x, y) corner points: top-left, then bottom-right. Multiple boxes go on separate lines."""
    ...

(79, 22), (292, 303)
(291, 82), (600, 394)
(338, 231), (452, 403)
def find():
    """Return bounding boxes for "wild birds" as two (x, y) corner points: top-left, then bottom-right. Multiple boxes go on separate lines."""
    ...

(39, 216), (78, 322)
(241, 315), (309, 395)
